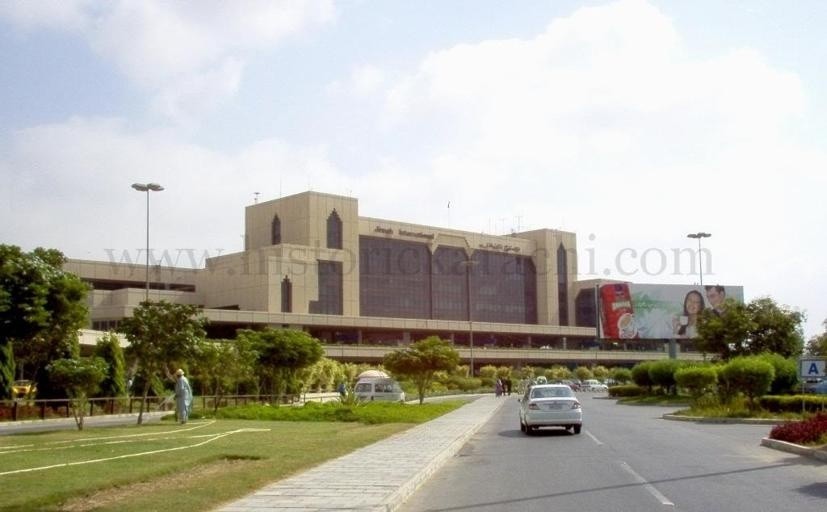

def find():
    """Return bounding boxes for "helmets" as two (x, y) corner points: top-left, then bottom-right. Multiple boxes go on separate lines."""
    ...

(176, 369), (185, 376)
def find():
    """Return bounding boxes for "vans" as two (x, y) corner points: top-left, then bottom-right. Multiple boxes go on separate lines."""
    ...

(353, 377), (405, 401)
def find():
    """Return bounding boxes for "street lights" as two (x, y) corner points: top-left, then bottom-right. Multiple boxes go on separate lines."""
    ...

(132, 183), (164, 300)
(461, 261), (479, 377)
(687, 232), (711, 285)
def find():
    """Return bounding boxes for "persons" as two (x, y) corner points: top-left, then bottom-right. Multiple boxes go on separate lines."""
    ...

(705, 285), (726, 318)
(671, 290), (705, 338)
(337, 381), (347, 399)
(496, 373), (512, 398)
(173, 368), (193, 424)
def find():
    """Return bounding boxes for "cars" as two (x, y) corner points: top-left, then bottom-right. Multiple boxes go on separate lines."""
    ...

(518, 377), (618, 434)
(806, 378), (827, 394)
(13, 380), (37, 398)
